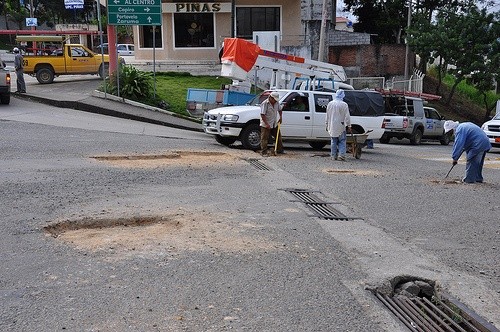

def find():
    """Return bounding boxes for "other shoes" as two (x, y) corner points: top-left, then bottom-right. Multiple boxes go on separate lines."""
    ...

(15, 90), (19, 93)
(331, 156), (337, 160)
(277, 150), (287, 155)
(339, 155), (346, 161)
(19, 90), (26, 93)
(260, 150), (267, 156)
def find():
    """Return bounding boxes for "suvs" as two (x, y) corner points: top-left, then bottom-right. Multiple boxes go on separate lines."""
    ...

(480, 112), (500, 148)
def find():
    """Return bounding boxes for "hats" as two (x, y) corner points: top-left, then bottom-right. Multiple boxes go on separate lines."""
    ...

(444, 120), (459, 134)
(14, 48), (19, 51)
(336, 89), (345, 100)
(270, 92), (280, 102)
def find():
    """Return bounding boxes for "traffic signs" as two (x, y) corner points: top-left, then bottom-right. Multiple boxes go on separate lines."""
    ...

(106, 0), (162, 26)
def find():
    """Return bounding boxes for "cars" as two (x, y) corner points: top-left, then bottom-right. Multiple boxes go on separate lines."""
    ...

(54, 47), (90, 56)
(117, 43), (134, 56)
(96, 43), (108, 54)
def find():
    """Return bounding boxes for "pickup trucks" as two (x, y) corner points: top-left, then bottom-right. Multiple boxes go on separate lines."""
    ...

(0, 58), (11, 104)
(21, 44), (126, 85)
(201, 89), (386, 149)
(379, 105), (452, 145)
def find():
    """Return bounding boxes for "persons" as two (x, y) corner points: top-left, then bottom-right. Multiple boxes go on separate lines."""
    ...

(14, 48), (26, 93)
(259, 92), (284, 156)
(326, 89), (353, 160)
(444, 120), (492, 184)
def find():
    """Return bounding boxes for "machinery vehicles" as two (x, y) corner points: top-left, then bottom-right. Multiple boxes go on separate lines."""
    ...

(186, 38), (355, 119)
